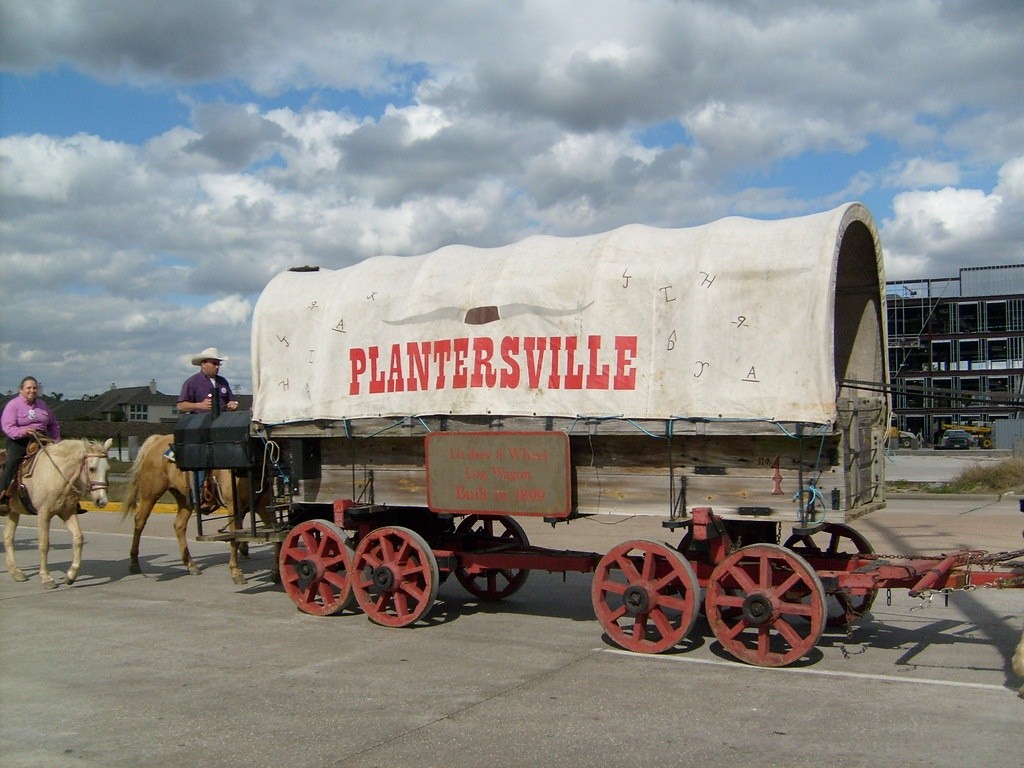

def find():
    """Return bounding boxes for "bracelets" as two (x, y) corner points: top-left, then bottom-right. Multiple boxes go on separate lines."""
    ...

(235, 401), (239, 405)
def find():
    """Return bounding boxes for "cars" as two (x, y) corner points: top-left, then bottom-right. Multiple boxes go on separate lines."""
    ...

(940, 429), (972, 450)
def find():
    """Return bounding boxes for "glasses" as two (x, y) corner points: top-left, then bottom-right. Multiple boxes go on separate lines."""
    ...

(206, 361), (222, 366)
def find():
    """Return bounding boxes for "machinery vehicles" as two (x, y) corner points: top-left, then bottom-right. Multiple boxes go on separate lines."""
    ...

(942, 423), (992, 448)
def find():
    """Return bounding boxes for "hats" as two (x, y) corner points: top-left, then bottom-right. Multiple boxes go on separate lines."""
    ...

(192, 348), (227, 366)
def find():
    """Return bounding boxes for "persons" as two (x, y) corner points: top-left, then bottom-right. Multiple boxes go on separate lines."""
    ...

(0, 377), (88, 517)
(176, 348), (239, 505)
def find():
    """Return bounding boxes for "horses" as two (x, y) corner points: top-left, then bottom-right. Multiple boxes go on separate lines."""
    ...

(0, 437), (114, 590)
(122, 433), (285, 585)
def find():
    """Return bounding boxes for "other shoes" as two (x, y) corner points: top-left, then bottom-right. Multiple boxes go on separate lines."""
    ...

(0, 502), (8, 517)
(200, 500), (210, 510)
(78, 508), (88, 514)
